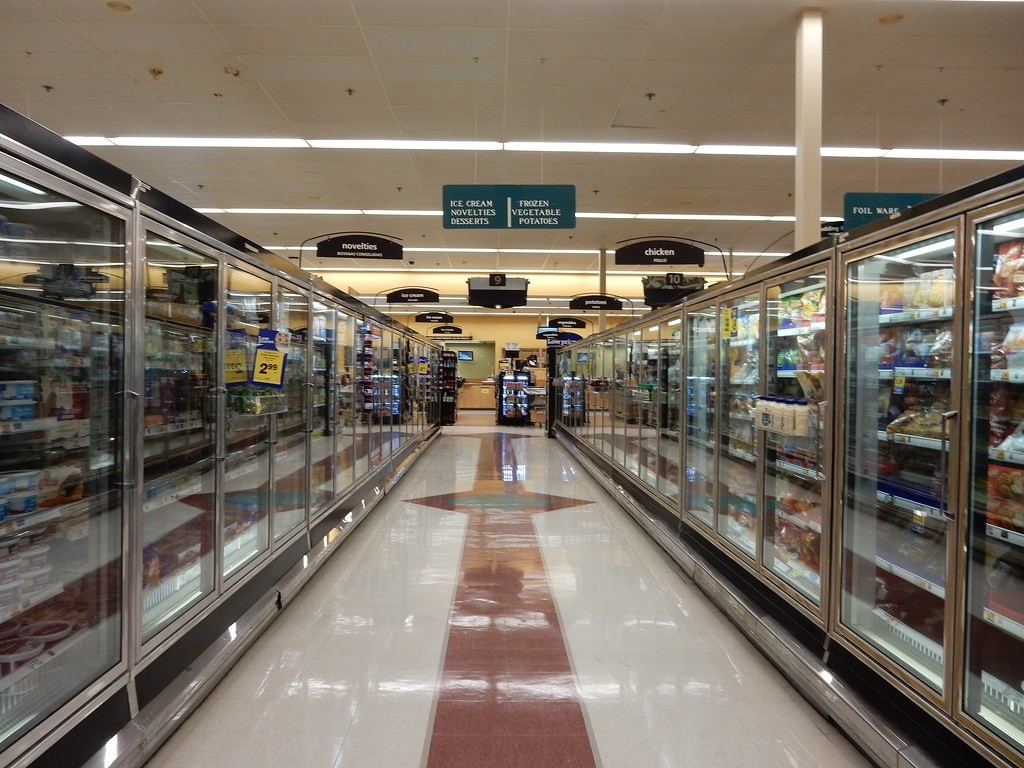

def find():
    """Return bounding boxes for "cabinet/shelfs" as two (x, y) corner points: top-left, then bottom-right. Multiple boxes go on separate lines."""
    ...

(224, 251), (273, 584)
(555, 294), (684, 507)
(0, 132), (136, 768)
(685, 298), (715, 538)
(966, 194), (1022, 768)
(272, 274), (310, 586)
(137, 214), (224, 680)
(310, 297), (441, 518)
(715, 281), (762, 571)
(837, 208), (965, 721)
(762, 259), (836, 622)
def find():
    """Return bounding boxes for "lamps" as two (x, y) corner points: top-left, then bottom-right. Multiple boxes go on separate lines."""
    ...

(641, 272), (707, 312)
(468, 278), (530, 310)
